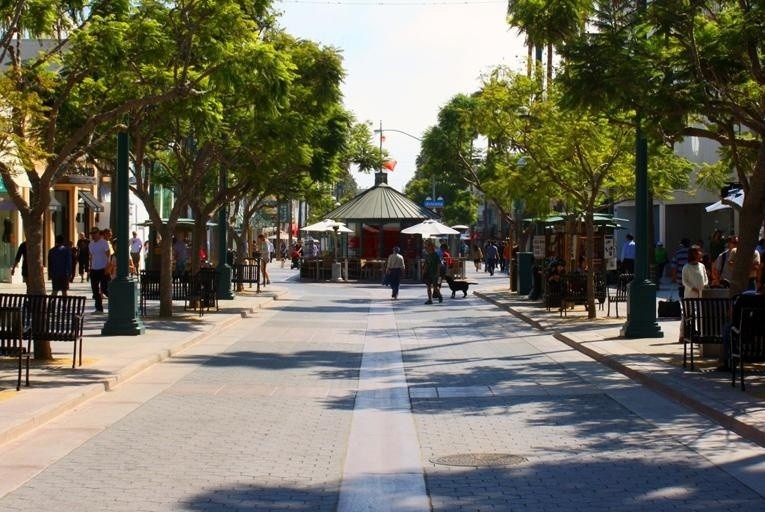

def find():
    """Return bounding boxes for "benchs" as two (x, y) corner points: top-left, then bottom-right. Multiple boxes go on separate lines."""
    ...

(0, 291), (90, 370)
(542, 269), (608, 310)
(725, 293), (765, 391)
(0, 304), (30, 391)
(134, 268), (225, 314)
(676, 295), (752, 371)
(228, 263), (261, 299)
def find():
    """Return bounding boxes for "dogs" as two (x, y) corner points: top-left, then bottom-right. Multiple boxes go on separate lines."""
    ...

(444, 274), (479, 299)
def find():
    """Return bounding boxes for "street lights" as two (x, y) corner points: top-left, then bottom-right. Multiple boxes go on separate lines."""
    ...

(374, 127), (437, 214)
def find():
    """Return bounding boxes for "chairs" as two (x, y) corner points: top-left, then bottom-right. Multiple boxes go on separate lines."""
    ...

(182, 274), (208, 317)
(300, 254), (465, 284)
(545, 275), (566, 315)
(606, 273), (636, 320)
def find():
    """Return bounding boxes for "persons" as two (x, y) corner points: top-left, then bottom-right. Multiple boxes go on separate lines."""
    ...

(418, 238), (520, 279)
(11, 226), (298, 315)
(679, 243), (709, 343)
(385, 246), (406, 301)
(653, 227), (765, 321)
(620, 232), (635, 274)
(717, 261), (765, 372)
(420, 240), (443, 305)
(576, 255), (588, 275)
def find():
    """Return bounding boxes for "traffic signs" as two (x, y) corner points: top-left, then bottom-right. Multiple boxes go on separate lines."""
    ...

(423, 199), (445, 209)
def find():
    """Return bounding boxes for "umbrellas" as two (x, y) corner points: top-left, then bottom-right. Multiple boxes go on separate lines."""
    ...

(298, 217), (355, 261)
(399, 218), (462, 257)
